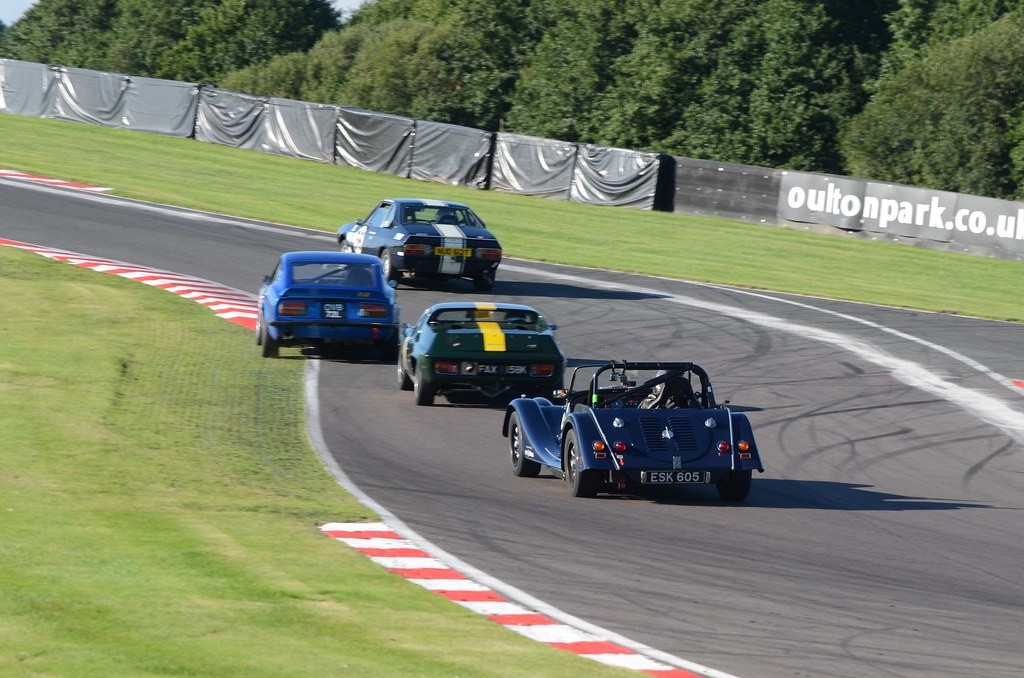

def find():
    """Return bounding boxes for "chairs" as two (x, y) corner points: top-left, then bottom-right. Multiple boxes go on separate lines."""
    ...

(648, 377), (700, 409)
(345, 268), (373, 286)
(436, 214), (459, 225)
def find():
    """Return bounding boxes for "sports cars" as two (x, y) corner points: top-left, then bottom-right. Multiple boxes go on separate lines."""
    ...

(336, 198), (502, 293)
(502, 359), (765, 502)
(398, 302), (565, 406)
(256, 251), (398, 364)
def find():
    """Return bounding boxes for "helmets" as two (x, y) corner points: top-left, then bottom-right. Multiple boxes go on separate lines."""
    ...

(350, 270), (370, 286)
(503, 312), (528, 322)
(655, 370), (684, 394)
(435, 208), (457, 224)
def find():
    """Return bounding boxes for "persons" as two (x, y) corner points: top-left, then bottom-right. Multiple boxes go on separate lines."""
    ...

(436, 209), (453, 223)
(638, 371), (668, 408)
